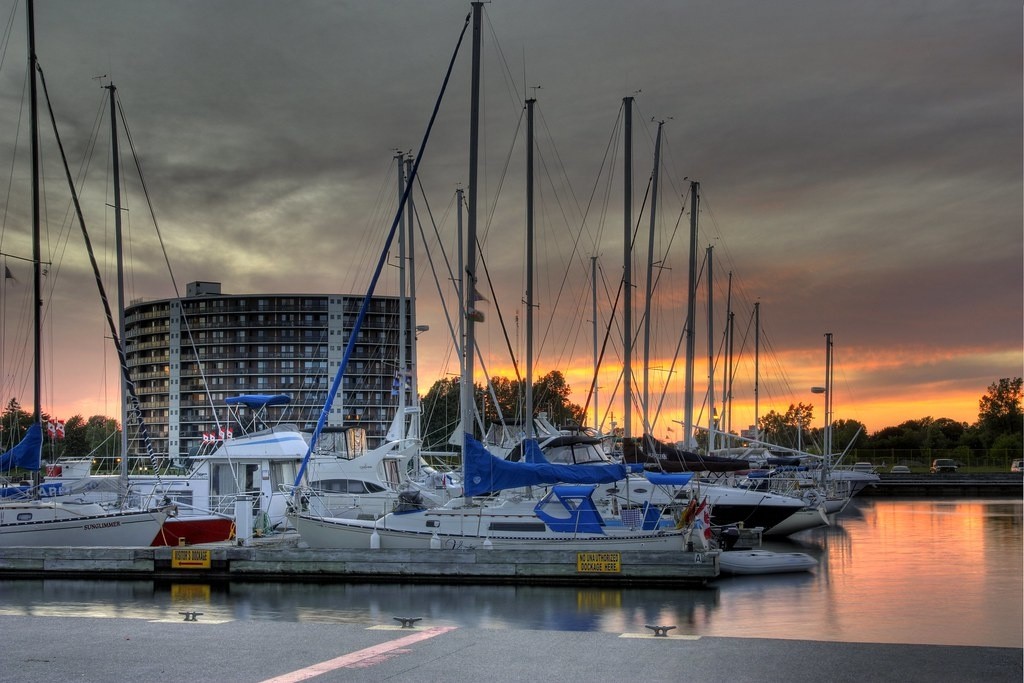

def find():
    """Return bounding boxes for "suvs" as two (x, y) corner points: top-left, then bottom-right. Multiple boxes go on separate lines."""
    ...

(930, 459), (957, 473)
(1011, 460), (1024, 471)
(853, 462), (873, 473)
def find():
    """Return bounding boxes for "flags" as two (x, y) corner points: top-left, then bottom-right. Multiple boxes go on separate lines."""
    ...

(227, 427), (232, 440)
(210, 432), (215, 444)
(48, 420), (65, 439)
(0, 424), (41, 472)
(203, 432), (209, 446)
(219, 427), (225, 442)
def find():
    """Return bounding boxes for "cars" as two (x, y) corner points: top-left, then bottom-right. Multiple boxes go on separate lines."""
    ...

(891, 465), (910, 473)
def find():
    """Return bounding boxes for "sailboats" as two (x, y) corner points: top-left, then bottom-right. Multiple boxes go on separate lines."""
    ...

(0, 1), (880, 552)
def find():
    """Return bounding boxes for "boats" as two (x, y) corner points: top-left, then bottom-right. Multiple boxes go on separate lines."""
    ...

(719, 550), (819, 574)
